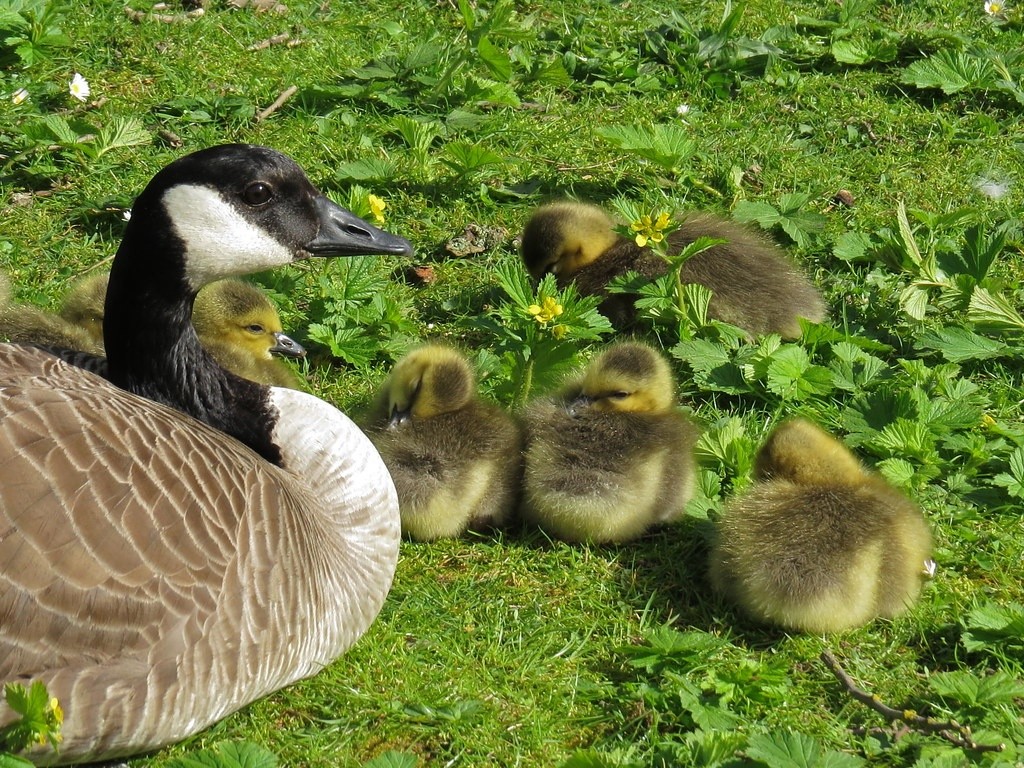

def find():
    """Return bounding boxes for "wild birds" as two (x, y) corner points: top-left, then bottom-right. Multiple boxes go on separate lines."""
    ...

(0, 142), (936, 768)
